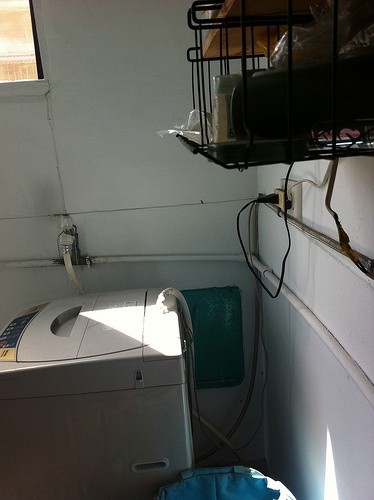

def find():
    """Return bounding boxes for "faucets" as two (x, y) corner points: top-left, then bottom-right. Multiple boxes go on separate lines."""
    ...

(56, 216), (78, 252)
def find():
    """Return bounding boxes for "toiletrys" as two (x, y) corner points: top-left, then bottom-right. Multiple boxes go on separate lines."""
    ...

(212, 74), (241, 145)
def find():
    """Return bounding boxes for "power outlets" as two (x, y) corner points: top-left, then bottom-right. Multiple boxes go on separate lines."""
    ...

(280, 178), (303, 219)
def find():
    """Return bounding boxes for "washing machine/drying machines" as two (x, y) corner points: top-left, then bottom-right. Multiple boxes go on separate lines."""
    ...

(0, 287), (199, 500)
